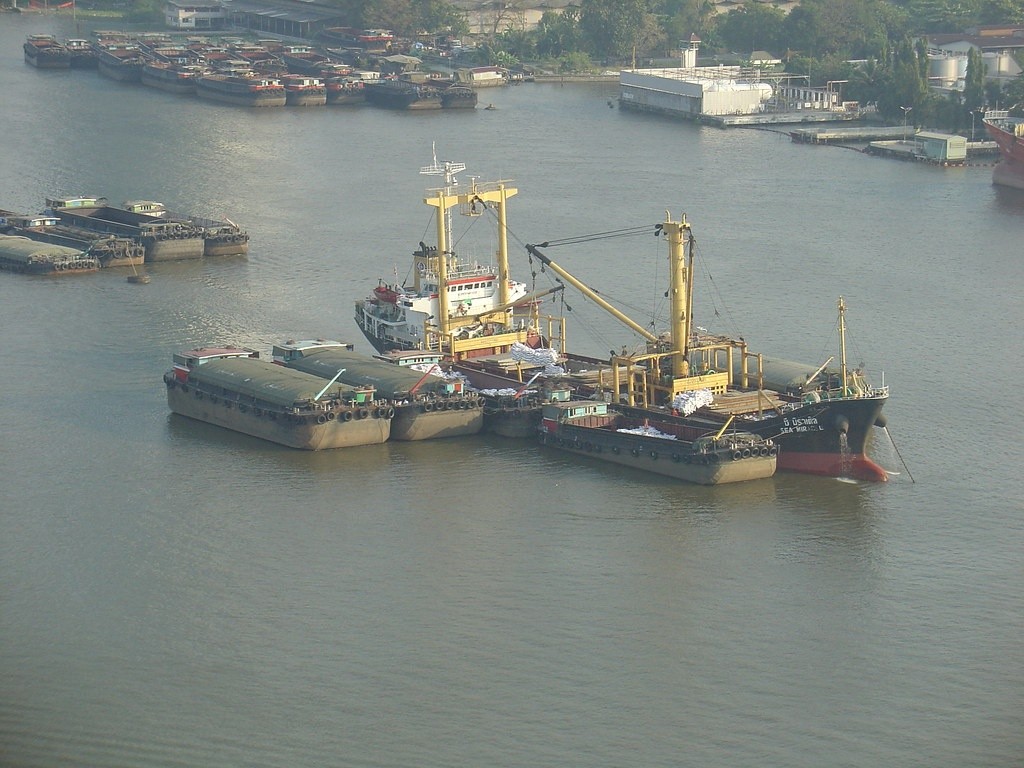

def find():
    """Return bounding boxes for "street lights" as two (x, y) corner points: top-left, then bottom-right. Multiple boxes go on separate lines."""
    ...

(969, 112), (975, 146)
(899, 106), (912, 139)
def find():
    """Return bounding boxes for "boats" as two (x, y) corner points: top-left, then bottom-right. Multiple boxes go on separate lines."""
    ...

(982, 116), (1024, 190)
(0, 233), (102, 276)
(122, 199), (250, 256)
(0, 210), (145, 267)
(371, 346), (574, 441)
(39, 194), (206, 262)
(354, 141), (893, 485)
(23, 25), (513, 111)
(272, 338), (487, 440)
(162, 343), (396, 451)
(534, 376), (779, 486)
